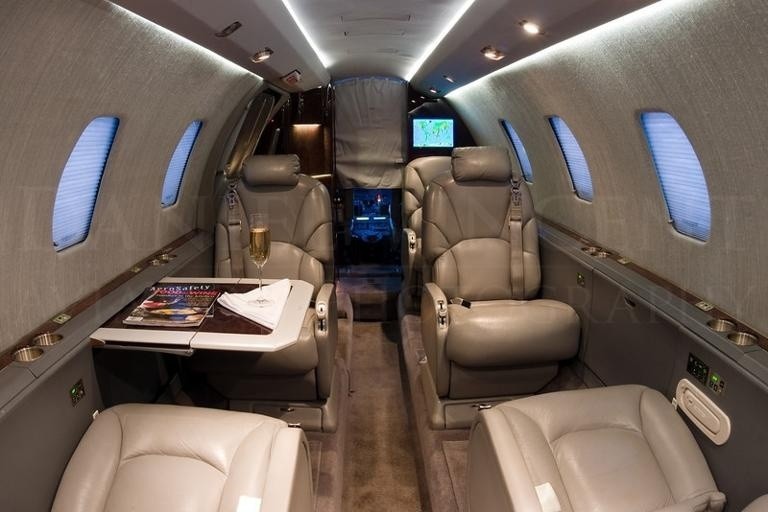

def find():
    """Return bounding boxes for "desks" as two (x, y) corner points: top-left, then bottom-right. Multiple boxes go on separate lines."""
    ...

(91, 276), (314, 358)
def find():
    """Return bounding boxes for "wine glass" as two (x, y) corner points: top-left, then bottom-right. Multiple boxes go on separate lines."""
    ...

(247, 212), (270, 308)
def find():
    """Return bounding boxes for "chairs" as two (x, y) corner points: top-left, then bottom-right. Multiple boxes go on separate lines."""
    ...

(52, 402), (315, 511)
(466, 383), (767, 512)
(205, 154), (340, 433)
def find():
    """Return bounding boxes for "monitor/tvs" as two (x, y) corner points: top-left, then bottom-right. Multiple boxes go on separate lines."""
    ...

(409, 116), (457, 152)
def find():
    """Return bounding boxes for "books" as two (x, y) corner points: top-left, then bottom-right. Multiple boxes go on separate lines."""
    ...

(121, 282), (221, 328)
